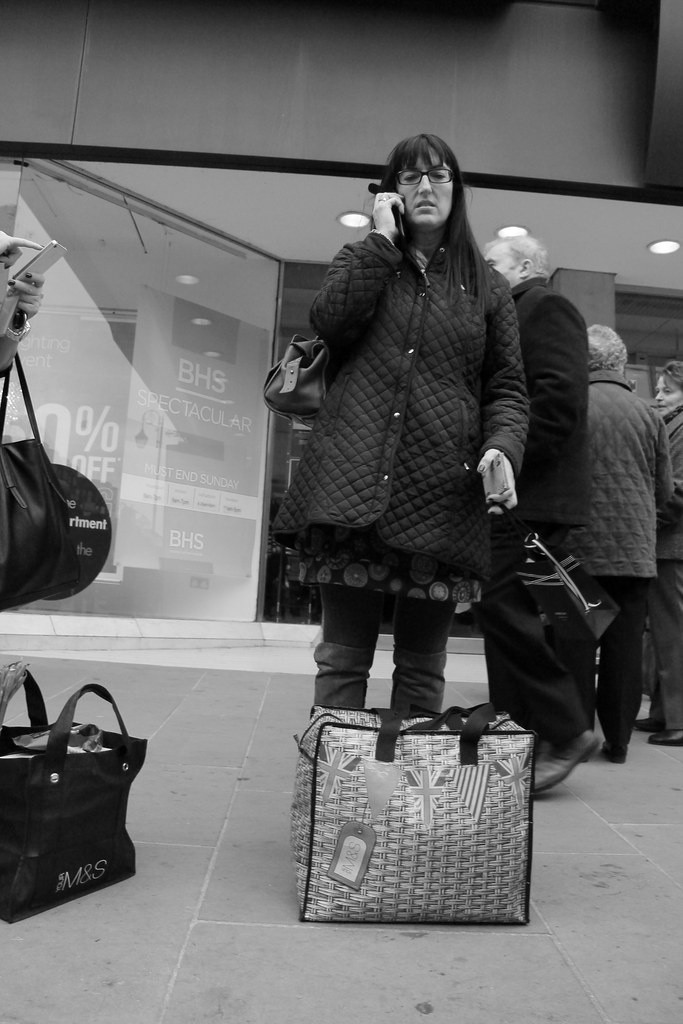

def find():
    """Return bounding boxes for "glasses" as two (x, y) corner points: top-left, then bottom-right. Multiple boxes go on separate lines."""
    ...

(397, 169), (455, 185)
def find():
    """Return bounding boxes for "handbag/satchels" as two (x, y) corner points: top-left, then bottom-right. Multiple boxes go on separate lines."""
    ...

(0, 660), (148, 924)
(263, 335), (338, 430)
(487, 502), (620, 650)
(0, 352), (84, 611)
(290, 700), (537, 925)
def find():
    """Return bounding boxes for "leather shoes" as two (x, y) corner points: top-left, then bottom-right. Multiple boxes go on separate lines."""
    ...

(634, 717), (664, 732)
(648, 730), (683, 746)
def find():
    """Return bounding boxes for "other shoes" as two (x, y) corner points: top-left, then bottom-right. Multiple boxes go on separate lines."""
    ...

(603, 741), (626, 763)
(533, 730), (600, 792)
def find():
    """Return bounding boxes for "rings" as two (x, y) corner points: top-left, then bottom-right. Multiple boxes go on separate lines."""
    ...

(378, 194), (389, 201)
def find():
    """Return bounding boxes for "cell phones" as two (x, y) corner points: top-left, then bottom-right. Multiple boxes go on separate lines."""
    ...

(482, 452), (509, 501)
(12, 239), (68, 285)
(397, 206), (405, 238)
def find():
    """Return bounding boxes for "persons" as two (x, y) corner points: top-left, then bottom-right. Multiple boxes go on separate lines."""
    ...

(0, 231), (46, 377)
(272, 135), (529, 713)
(587, 324), (674, 764)
(634, 361), (683, 745)
(484, 235), (603, 795)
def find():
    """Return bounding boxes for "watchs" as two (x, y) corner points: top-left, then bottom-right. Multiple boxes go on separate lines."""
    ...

(6, 322), (31, 342)
